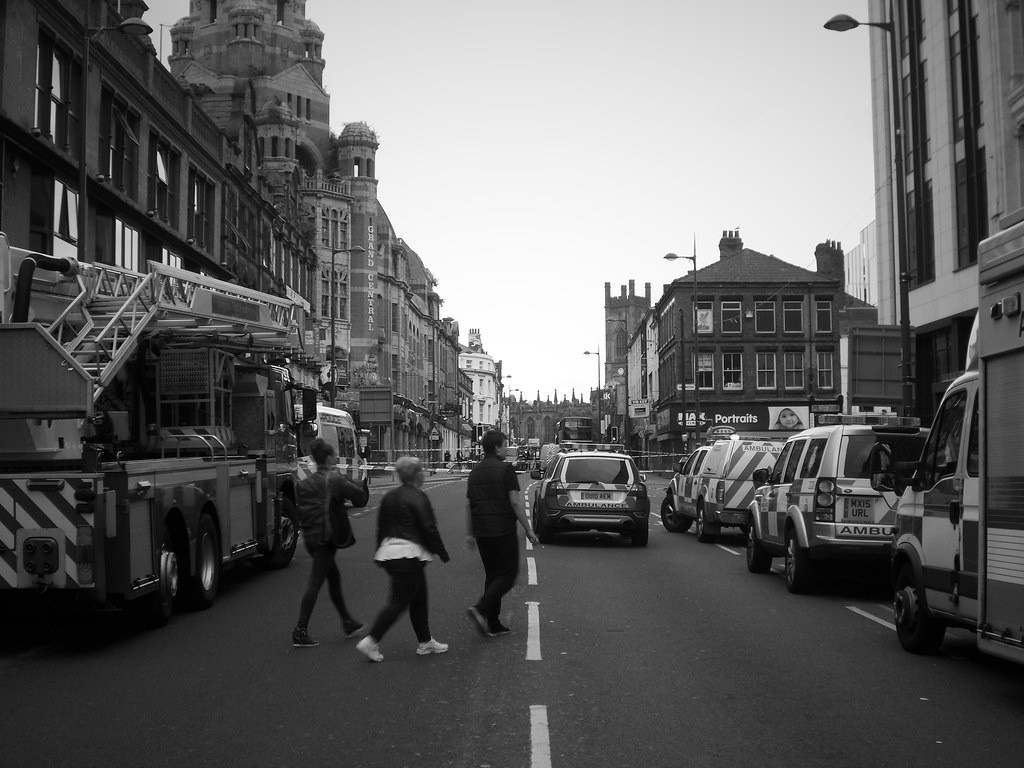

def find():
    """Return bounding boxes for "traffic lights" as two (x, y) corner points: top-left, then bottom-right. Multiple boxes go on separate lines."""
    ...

(612, 427), (617, 441)
(477, 427), (483, 441)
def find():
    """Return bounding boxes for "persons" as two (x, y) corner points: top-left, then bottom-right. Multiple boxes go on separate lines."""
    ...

(356, 455), (449, 662)
(467, 431), (539, 636)
(456, 450), (463, 465)
(773, 409), (804, 430)
(444, 450), (450, 465)
(293, 438), (368, 647)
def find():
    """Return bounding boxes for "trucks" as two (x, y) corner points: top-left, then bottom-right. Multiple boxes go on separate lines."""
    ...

(294, 400), (371, 507)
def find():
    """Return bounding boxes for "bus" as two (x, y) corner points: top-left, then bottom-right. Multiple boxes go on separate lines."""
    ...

(555, 417), (593, 443)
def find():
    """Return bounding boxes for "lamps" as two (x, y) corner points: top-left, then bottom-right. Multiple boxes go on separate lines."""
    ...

(145, 209), (155, 217)
(31, 127), (41, 137)
(96, 174), (105, 182)
(220, 260), (227, 267)
(186, 237), (194, 245)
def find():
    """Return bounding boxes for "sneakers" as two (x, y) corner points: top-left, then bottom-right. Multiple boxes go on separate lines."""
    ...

(292, 629), (319, 646)
(356, 636), (384, 662)
(344, 621), (366, 638)
(465, 603), (489, 636)
(488, 620), (511, 636)
(417, 636), (448, 655)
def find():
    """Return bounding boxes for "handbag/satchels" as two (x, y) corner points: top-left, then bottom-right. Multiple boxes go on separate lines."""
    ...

(324, 471), (356, 548)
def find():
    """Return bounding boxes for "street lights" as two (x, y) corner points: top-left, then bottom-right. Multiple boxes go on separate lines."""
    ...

(605, 316), (631, 455)
(664, 252), (702, 449)
(823, 14), (915, 415)
(508, 388), (519, 446)
(331, 245), (366, 407)
(499, 375), (512, 430)
(584, 351), (602, 444)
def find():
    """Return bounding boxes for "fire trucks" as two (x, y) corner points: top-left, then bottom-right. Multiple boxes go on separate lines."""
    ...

(0, 230), (337, 628)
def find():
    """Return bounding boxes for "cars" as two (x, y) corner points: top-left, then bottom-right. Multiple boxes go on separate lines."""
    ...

(502, 444), (650, 546)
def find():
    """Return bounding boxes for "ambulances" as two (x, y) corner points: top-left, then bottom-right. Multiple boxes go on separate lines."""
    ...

(746, 414), (931, 595)
(870, 222), (1024, 664)
(661, 439), (786, 543)
